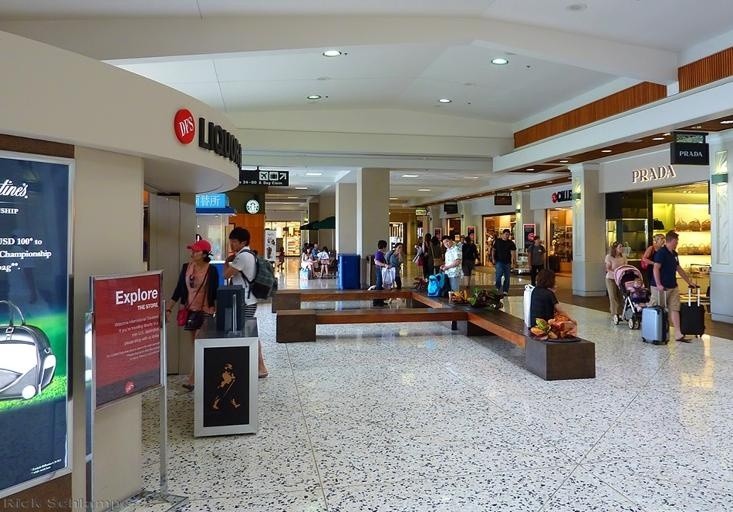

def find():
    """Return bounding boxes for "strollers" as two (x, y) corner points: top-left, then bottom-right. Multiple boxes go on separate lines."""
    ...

(612, 263), (651, 332)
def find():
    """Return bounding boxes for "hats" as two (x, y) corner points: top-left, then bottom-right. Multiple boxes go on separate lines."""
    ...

(187, 239), (212, 254)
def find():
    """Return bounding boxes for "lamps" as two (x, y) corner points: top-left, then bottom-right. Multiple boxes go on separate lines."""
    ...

(572, 193), (581, 200)
(711, 174), (728, 185)
(515, 208), (520, 214)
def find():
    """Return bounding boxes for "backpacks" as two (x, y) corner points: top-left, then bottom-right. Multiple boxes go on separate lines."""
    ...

(239, 249), (275, 301)
(427, 269), (451, 297)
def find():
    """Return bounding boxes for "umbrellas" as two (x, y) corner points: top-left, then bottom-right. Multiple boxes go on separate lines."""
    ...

(299, 220), (321, 245)
(313, 216), (335, 249)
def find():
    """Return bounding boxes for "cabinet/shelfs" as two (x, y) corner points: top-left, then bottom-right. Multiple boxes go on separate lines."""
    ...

(651, 224), (711, 296)
(549, 225), (572, 263)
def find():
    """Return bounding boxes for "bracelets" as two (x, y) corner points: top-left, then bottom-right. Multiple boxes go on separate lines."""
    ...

(164, 309), (171, 312)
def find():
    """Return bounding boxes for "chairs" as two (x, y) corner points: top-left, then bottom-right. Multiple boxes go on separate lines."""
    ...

(297, 248), (338, 281)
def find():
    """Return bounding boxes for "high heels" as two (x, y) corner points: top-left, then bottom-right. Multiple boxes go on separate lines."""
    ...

(182, 382), (197, 393)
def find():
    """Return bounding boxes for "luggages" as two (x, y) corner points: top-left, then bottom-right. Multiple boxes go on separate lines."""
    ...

(679, 285), (706, 338)
(641, 288), (669, 345)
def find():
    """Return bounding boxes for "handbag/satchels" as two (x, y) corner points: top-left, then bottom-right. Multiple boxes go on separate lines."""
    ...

(381, 266), (396, 290)
(183, 309), (205, 331)
(177, 307), (187, 327)
(416, 255), (424, 266)
(0, 300), (56, 402)
(675, 217), (711, 256)
(640, 246), (655, 270)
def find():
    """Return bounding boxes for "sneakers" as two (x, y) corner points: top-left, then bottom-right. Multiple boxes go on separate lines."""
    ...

(252, 370), (269, 378)
(503, 291), (508, 296)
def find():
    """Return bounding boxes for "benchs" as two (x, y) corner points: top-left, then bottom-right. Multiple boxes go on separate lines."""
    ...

(411, 286), (596, 380)
(269, 285), (468, 343)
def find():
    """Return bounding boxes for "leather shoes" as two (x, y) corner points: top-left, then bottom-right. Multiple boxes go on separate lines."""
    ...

(675, 335), (693, 342)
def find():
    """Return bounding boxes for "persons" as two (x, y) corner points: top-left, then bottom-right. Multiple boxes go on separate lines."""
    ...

(221, 227), (269, 377)
(490, 228), (516, 296)
(648, 231), (697, 343)
(210, 364), (241, 412)
(604, 241), (628, 324)
(626, 278), (648, 312)
(532, 267), (579, 337)
(277, 246), (285, 270)
(299, 241), (337, 280)
(526, 236), (545, 284)
(372, 229), (479, 306)
(641, 234), (665, 297)
(164, 240), (218, 390)
